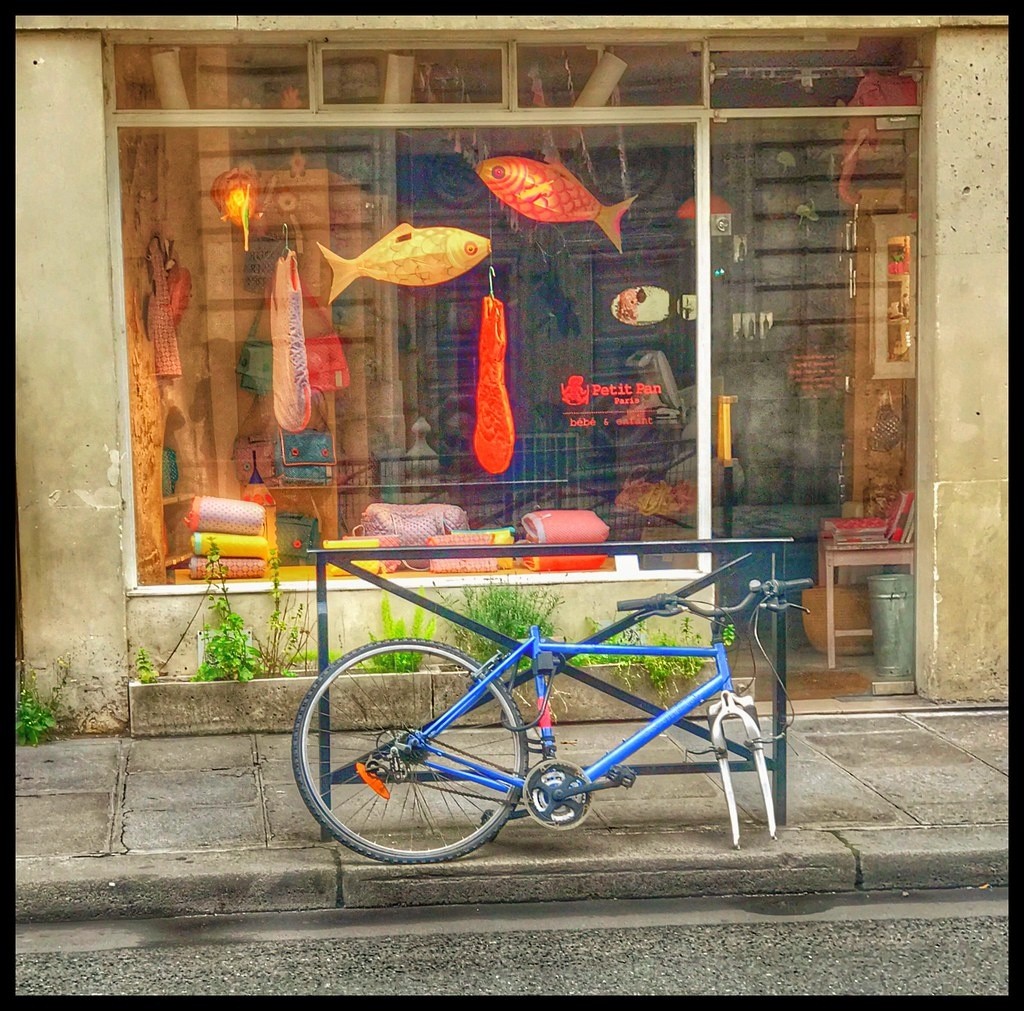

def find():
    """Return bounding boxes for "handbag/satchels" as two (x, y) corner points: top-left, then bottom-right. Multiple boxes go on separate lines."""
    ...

(298, 277), (349, 394)
(235, 435), (276, 483)
(274, 426), (336, 483)
(234, 293), (276, 398)
(275, 512), (319, 557)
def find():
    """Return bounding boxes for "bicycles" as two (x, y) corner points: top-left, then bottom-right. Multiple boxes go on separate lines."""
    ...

(288, 575), (816, 863)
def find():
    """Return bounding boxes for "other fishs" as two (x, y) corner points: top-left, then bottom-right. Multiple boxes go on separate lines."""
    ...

(313, 222), (491, 306)
(475, 158), (639, 257)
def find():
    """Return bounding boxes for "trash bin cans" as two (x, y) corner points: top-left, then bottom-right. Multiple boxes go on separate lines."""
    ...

(867, 573), (913, 677)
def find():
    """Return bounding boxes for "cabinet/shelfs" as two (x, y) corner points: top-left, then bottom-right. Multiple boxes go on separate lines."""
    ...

(865, 213), (916, 379)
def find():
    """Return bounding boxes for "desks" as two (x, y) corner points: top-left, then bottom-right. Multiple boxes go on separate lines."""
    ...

(817, 536), (917, 667)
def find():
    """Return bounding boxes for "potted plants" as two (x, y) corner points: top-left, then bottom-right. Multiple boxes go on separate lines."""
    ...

(128, 537), (738, 738)
